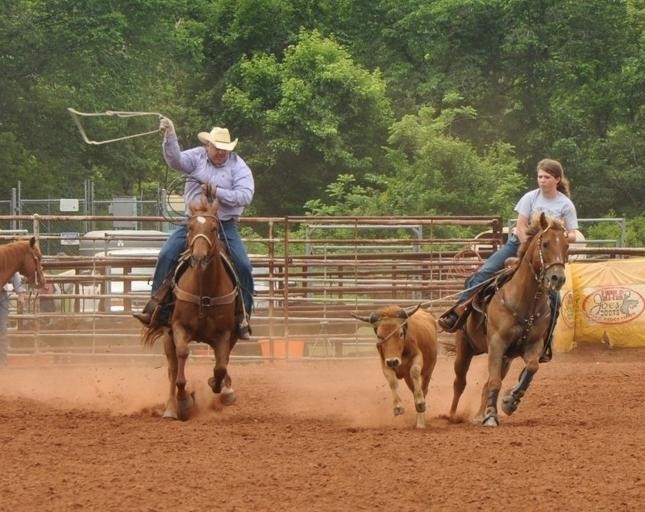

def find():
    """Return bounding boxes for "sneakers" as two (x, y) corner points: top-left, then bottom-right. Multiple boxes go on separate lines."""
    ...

(239, 330), (250, 340)
(539, 354), (549, 362)
(134, 312), (165, 326)
(438, 314), (457, 329)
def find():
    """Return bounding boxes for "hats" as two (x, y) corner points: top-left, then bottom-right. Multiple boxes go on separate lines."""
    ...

(197, 126), (239, 151)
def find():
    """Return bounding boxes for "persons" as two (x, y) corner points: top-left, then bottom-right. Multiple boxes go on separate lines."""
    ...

(443, 159), (579, 328)
(0, 272), (26, 368)
(133, 116), (256, 340)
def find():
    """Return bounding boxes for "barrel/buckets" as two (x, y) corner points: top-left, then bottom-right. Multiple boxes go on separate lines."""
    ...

(287, 339), (305, 357)
(257, 339), (287, 369)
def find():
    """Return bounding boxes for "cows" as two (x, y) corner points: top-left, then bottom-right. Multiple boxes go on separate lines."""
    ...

(347, 302), (439, 430)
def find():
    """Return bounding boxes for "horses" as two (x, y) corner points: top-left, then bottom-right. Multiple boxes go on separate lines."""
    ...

(448, 210), (571, 429)
(139, 198), (244, 421)
(0, 234), (46, 295)
